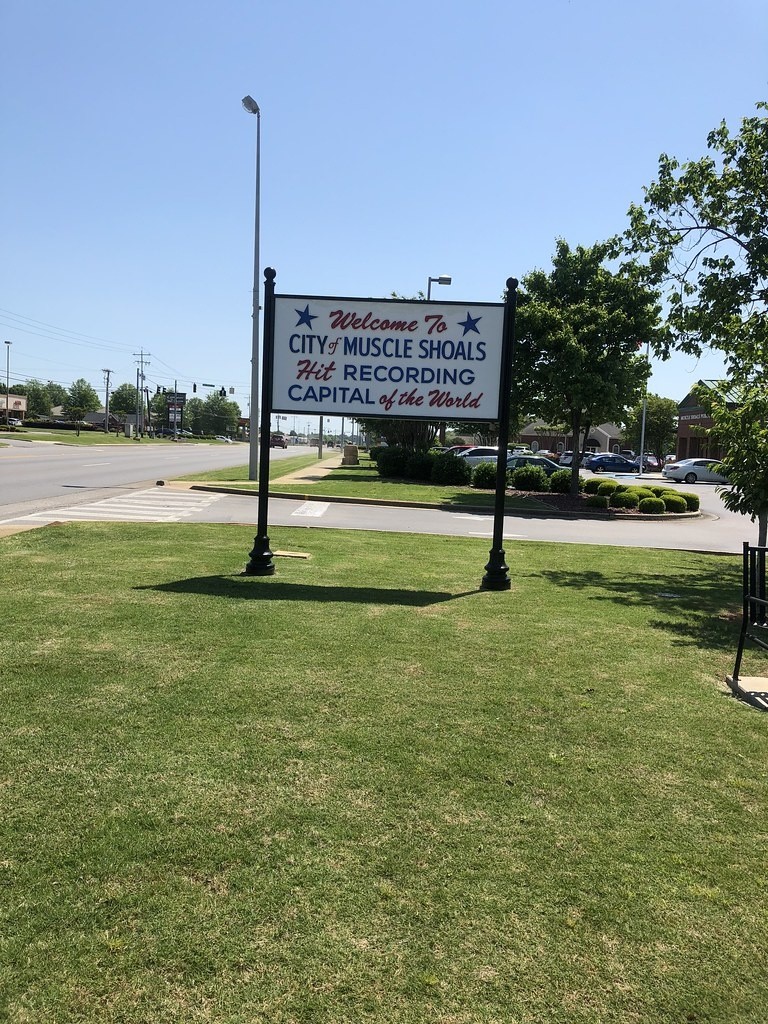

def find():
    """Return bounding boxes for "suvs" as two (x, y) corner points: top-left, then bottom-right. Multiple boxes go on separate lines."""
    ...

(310, 438), (320, 447)
(270, 435), (289, 450)
(327, 441), (335, 448)
(559, 450), (594, 467)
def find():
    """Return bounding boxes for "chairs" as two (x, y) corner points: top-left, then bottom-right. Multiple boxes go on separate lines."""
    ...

(733, 541), (768, 686)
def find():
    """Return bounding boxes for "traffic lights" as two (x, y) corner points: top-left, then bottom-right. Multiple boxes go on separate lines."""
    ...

(156, 385), (161, 394)
(163, 386), (167, 395)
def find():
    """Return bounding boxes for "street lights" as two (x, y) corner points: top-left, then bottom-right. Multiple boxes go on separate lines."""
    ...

(4, 341), (13, 425)
(426, 275), (453, 300)
(241, 93), (261, 486)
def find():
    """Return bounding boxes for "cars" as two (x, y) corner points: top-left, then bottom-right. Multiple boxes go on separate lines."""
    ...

(662, 458), (734, 485)
(513, 446), (533, 455)
(538, 450), (554, 456)
(584, 454), (645, 474)
(445, 445), (478, 457)
(457, 447), (513, 466)
(154, 428), (178, 435)
(215, 435), (233, 444)
(8, 418), (22, 425)
(507, 455), (568, 478)
(175, 428), (192, 435)
(636, 456), (658, 471)
(622, 450), (637, 459)
(666, 454), (677, 462)
